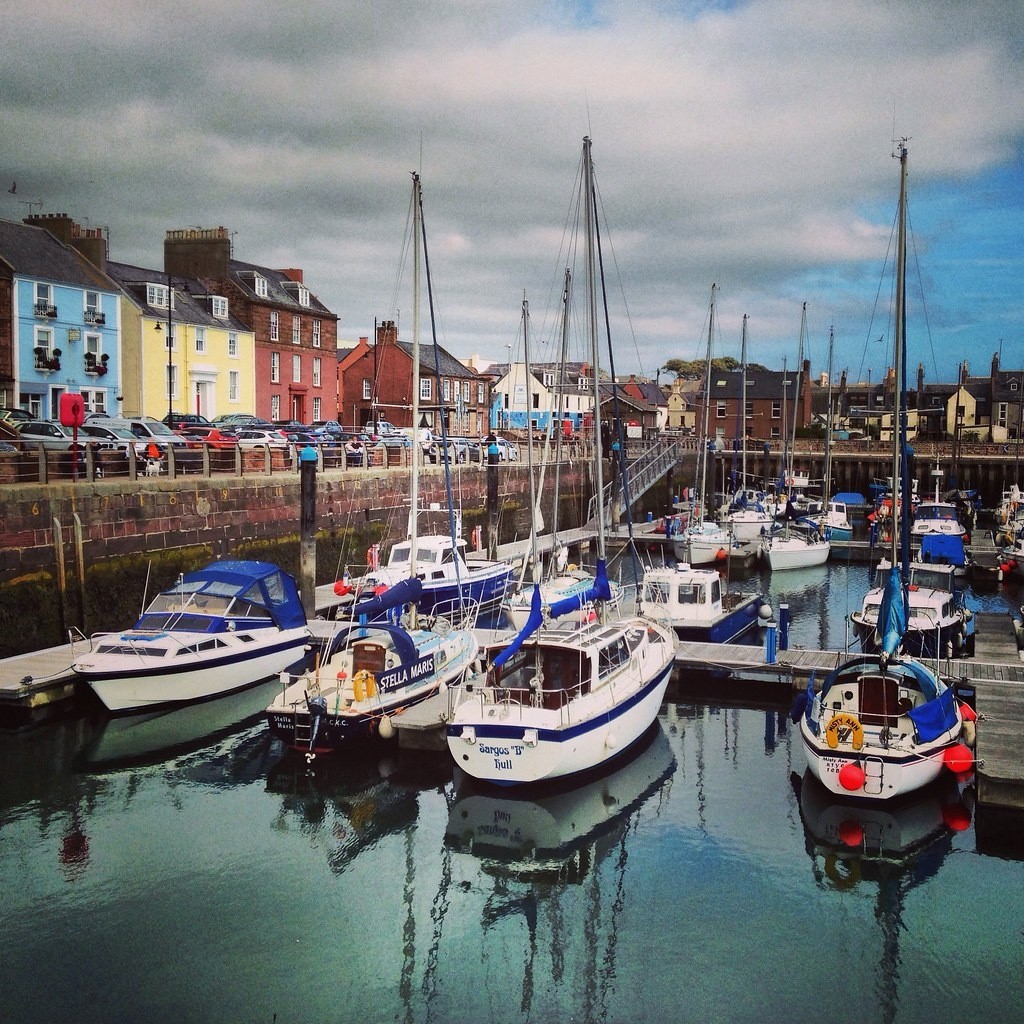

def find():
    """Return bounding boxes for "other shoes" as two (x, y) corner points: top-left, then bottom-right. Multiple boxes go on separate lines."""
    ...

(137, 472), (144, 476)
(160, 468), (163, 472)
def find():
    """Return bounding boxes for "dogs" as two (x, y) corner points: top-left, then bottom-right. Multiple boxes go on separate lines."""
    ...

(145, 459), (160, 477)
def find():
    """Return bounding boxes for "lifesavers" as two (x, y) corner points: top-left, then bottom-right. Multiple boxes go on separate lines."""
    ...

(365, 547), (382, 567)
(786, 478), (794, 485)
(567, 564), (577, 573)
(471, 529), (482, 547)
(826, 713), (865, 750)
(353, 670), (375, 702)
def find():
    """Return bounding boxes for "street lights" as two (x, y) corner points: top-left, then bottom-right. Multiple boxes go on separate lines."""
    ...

(363, 351), (377, 434)
(154, 321), (173, 429)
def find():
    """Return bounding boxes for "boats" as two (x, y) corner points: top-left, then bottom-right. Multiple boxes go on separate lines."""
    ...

(72, 561), (312, 711)
(74, 677), (290, 770)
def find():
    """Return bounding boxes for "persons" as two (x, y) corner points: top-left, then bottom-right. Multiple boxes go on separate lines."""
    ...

(345, 435), (372, 467)
(683, 486), (689, 501)
(83, 439), (103, 478)
(126, 439), (144, 476)
(689, 484), (694, 501)
(486, 432), (496, 446)
(144, 438), (166, 472)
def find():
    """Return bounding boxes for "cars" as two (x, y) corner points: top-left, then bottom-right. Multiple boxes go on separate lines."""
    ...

(83, 412), (111, 423)
(286, 431), (336, 454)
(274, 420), (308, 432)
(212, 414), (273, 430)
(335, 432), (379, 448)
(80, 424), (146, 452)
(0, 408), (37, 421)
(310, 420), (343, 435)
(172, 430), (203, 449)
(479, 435), (517, 462)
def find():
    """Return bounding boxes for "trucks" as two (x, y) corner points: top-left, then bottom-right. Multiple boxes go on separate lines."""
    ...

(86, 418), (188, 449)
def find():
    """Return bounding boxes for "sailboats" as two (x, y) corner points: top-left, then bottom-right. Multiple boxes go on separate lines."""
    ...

(790, 766), (976, 1024)
(266, 754), (421, 1024)
(268, 137), (1024, 793)
(444, 717), (676, 1024)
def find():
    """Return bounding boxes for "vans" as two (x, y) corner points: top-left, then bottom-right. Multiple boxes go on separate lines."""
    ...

(366, 421), (393, 435)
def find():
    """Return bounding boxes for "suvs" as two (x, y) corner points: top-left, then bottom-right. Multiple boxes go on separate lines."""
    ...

(429, 438), (481, 464)
(162, 412), (212, 429)
(13, 421), (117, 450)
(182, 427), (239, 449)
(235, 430), (290, 449)
